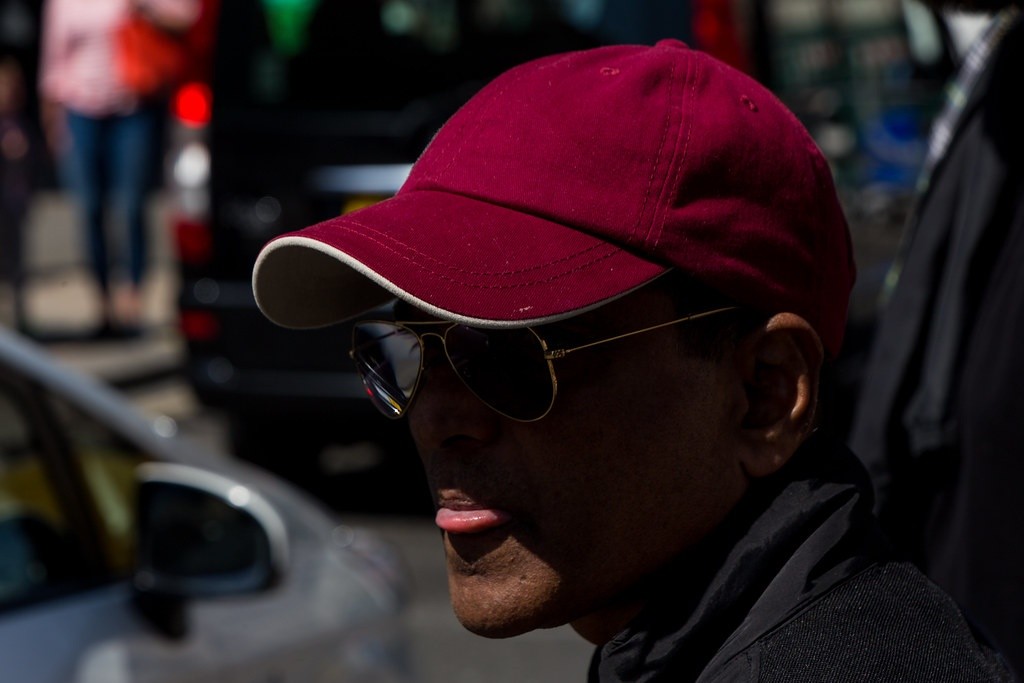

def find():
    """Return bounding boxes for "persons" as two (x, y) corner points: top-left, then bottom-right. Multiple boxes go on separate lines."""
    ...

(41, 0), (212, 320)
(252, 38), (1024, 683)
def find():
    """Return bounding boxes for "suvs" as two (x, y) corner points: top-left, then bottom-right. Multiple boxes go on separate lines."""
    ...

(164, 0), (967, 503)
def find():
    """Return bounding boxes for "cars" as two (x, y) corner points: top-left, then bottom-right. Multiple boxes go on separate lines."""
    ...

(0, 315), (425, 683)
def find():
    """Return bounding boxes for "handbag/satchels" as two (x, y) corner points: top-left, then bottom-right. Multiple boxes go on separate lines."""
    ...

(111, 0), (218, 98)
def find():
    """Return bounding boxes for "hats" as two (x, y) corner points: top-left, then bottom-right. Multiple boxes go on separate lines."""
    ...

(250, 40), (858, 363)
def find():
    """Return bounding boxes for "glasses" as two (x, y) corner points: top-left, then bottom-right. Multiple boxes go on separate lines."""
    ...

(349, 302), (761, 421)
